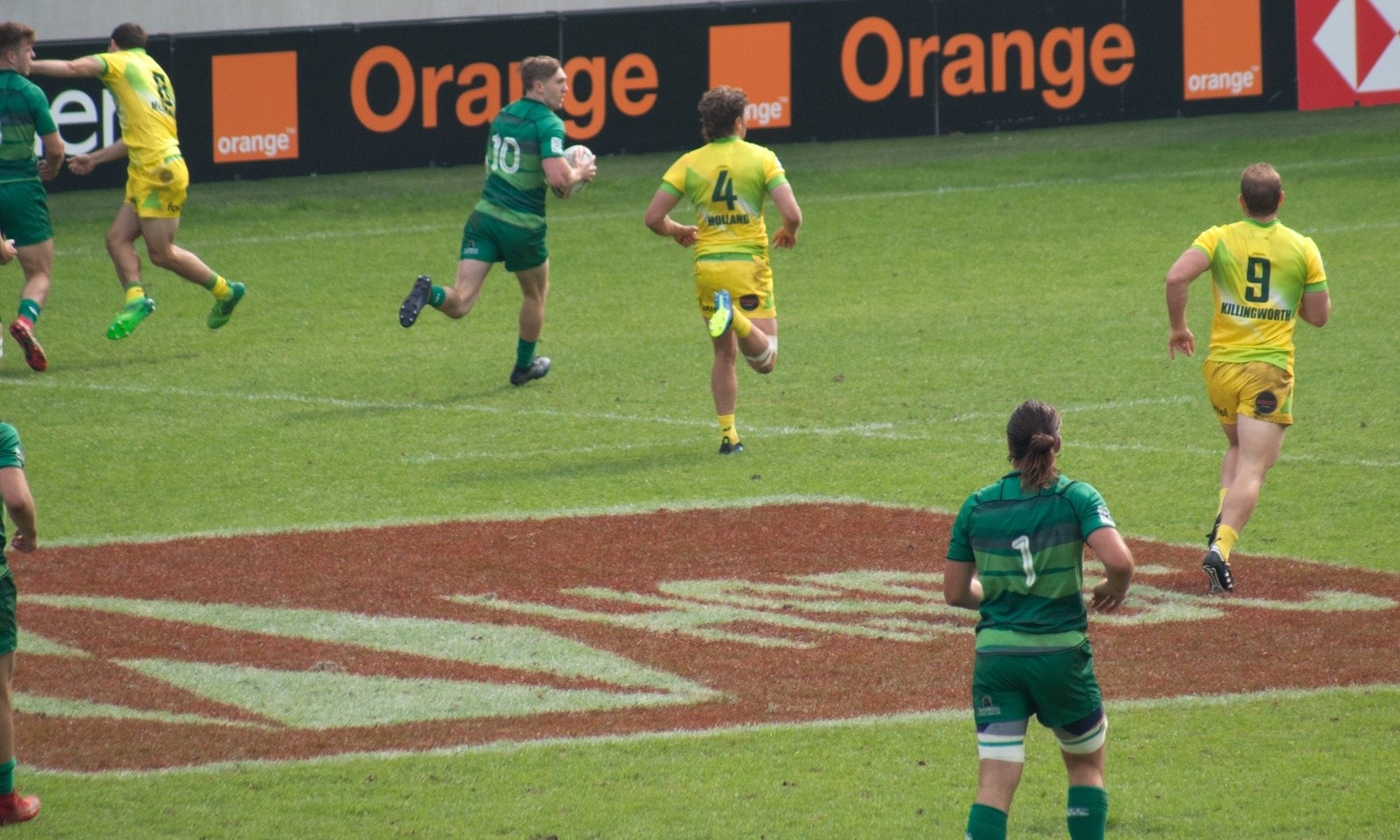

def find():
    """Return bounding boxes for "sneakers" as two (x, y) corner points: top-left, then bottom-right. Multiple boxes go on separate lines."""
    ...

(0, 789), (40, 825)
(1206, 513), (1222, 546)
(510, 357), (551, 385)
(720, 437), (743, 454)
(207, 280), (246, 329)
(10, 316), (47, 371)
(708, 289), (733, 338)
(399, 276), (433, 328)
(1202, 546), (1233, 599)
(106, 295), (156, 340)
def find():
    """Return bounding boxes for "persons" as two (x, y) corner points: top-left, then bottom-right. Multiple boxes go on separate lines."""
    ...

(1166, 162), (1331, 593)
(645, 86), (802, 454)
(0, 21), (65, 371)
(399, 57), (597, 386)
(944, 400), (1133, 840)
(0, 419), (41, 826)
(30, 22), (246, 339)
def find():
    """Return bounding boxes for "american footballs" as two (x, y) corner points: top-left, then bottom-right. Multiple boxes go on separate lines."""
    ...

(551, 144), (595, 199)
